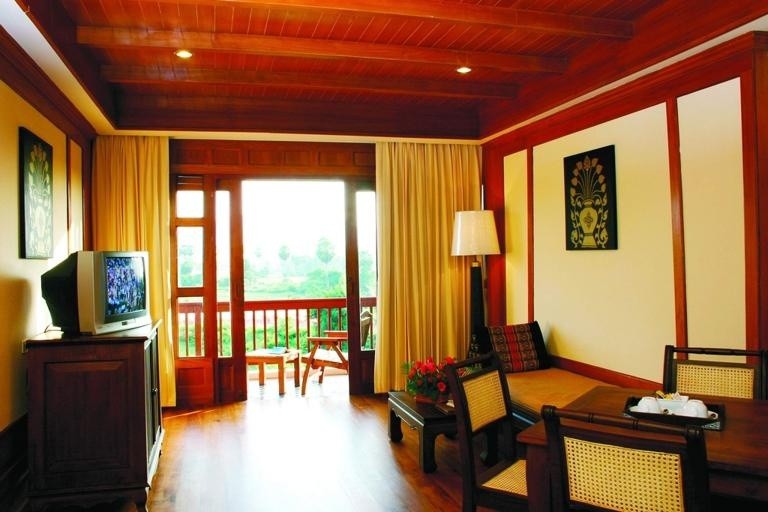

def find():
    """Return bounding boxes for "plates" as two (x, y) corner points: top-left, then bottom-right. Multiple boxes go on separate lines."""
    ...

(707, 410), (719, 419)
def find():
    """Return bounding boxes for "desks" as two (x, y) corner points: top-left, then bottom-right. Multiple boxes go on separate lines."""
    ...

(244, 349), (300, 396)
(516, 385), (768, 512)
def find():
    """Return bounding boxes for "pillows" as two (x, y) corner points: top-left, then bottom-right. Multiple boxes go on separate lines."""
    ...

(466, 320), (550, 373)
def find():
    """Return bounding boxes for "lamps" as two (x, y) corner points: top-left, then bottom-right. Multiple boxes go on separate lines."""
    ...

(450, 210), (502, 334)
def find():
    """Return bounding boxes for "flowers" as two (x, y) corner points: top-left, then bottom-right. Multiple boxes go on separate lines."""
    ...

(399, 356), (455, 401)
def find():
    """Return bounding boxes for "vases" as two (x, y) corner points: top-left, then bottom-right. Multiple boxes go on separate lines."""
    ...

(415, 394), (436, 405)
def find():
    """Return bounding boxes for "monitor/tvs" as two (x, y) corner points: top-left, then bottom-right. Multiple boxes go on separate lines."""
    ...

(40, 251), (152, 336)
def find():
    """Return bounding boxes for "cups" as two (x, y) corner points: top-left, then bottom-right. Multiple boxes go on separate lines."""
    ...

(630, 395), (708, 418)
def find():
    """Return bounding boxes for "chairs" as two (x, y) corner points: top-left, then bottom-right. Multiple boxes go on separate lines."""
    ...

(445, 351), (532, 512)
(301, 310), (373, 396)
(662, 345), (767, 400)
(541, 402), (714, 511)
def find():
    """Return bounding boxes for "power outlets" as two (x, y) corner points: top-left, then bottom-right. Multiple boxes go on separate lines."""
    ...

(21, 338), (30, 354)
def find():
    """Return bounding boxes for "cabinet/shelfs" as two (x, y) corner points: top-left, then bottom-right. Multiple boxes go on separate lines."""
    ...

(27, 318), (166, 512)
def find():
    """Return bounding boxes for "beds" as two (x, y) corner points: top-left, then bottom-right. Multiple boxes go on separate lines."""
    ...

(466, 322), (621, 457)
